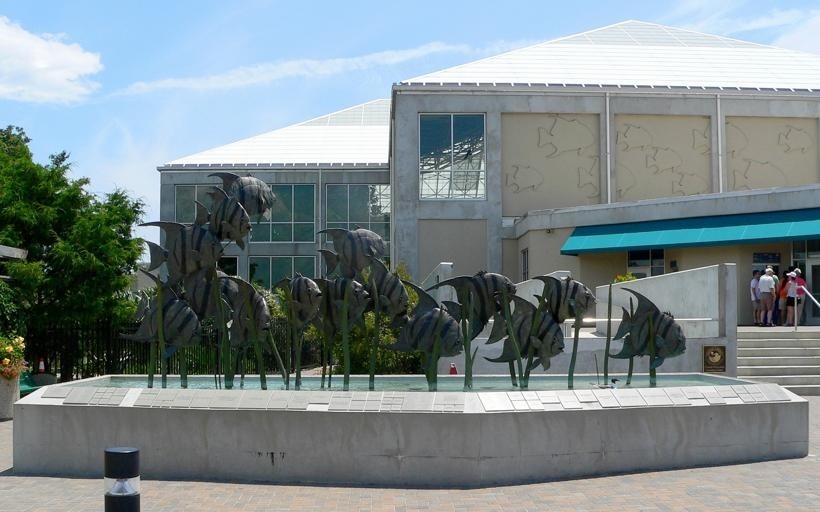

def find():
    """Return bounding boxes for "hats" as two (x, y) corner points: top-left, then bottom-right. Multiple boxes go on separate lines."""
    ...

(786, 268), (801, 278)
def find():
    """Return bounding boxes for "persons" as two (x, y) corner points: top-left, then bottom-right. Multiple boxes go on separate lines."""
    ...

(750, 265), (807, 326)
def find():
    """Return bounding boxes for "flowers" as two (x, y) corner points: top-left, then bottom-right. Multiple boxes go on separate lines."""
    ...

(0, 329), (30, 380)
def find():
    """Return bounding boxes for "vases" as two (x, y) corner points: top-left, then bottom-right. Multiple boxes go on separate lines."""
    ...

(0, 374), (20, 419)
